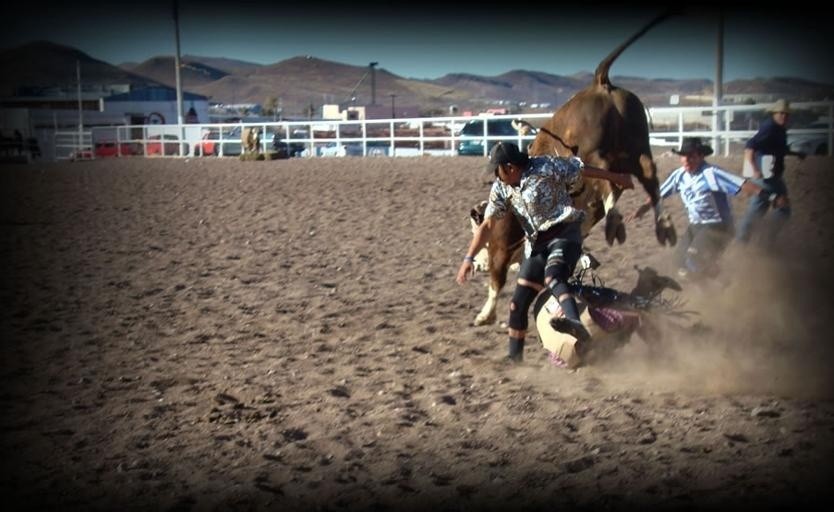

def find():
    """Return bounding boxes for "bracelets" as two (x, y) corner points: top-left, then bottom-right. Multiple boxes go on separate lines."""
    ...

(461, 254), (476, 263)
(638, 201), (651, 214)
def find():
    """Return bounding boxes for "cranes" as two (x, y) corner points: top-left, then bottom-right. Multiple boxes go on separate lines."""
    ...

(347, 62), (382, 107)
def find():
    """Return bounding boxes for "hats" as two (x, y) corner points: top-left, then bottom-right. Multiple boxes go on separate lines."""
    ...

(767, 98), (793, 115)
(672, 137), (714, 156)
(486, 143), (519, 174)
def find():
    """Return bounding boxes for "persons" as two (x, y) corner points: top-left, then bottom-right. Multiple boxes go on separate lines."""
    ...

(622, 135), (793, 294)
(453, 139), (637, 368)
(728, 93), (809, 262)
(184, 107), (198, 123)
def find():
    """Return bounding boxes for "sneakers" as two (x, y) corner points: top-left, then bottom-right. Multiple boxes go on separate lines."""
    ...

(550, 316), (592, 344)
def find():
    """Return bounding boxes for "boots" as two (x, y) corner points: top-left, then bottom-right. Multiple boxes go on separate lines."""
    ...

(634, 264), (682, 299)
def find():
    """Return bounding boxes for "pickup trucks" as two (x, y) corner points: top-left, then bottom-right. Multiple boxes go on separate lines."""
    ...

(220, 126), (306, 157)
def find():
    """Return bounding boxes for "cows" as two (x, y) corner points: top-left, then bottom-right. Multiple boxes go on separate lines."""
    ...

(469, 15), (677, 327)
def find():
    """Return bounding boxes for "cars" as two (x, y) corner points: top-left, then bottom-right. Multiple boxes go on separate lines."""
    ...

(195, 129), (229, 154)
(145, 136), (189, 157)
(73, 139), (130, 157)
(790, 122), (834, 157)
(456, 118), (535, 155)
